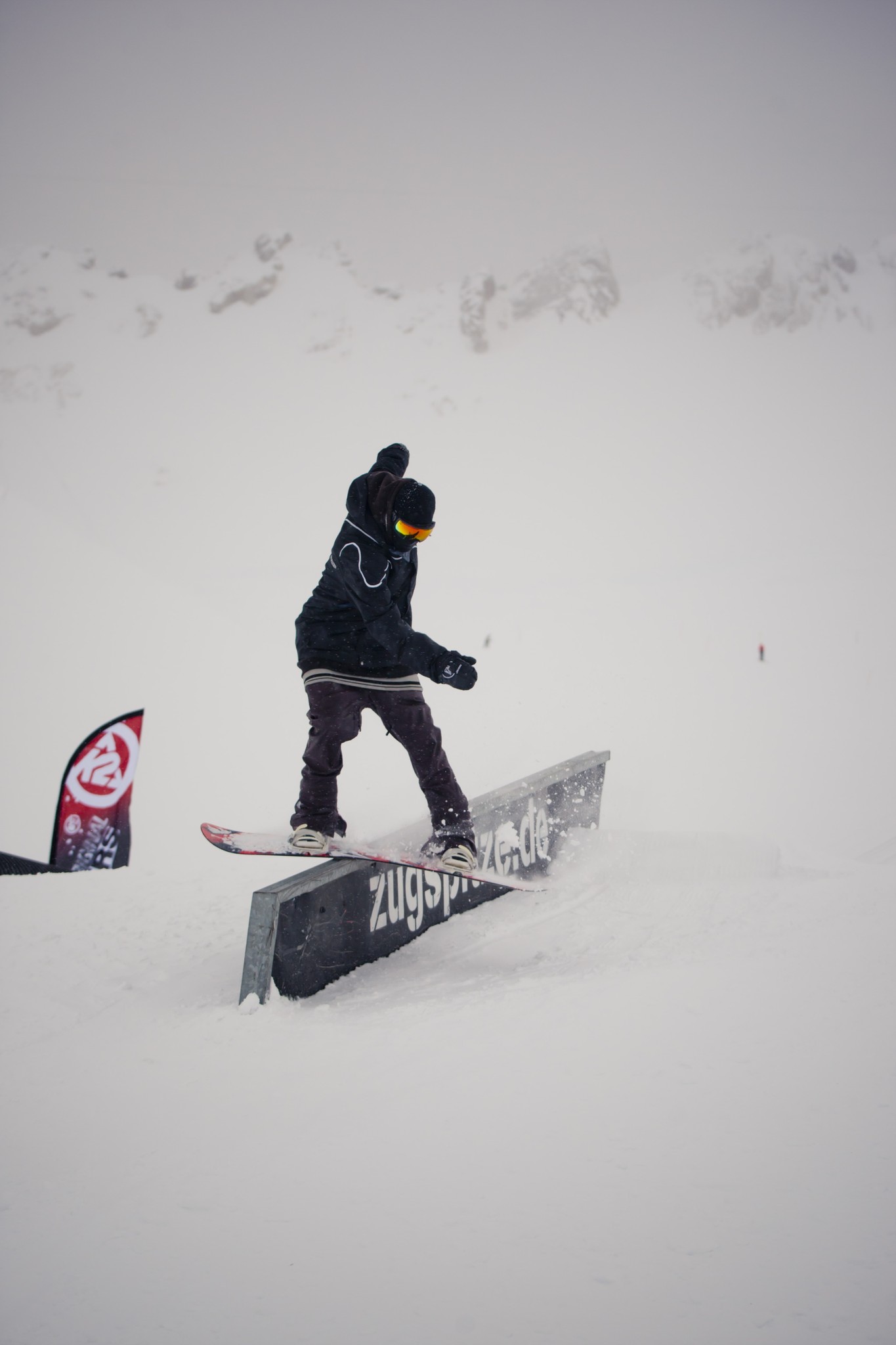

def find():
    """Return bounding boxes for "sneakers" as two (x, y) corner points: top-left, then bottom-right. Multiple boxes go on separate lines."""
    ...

(283, 822), (331, 856)
(438, 845), (478, 875)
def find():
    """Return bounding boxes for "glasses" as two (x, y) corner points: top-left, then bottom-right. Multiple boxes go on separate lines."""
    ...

(390, 511), (436, 543)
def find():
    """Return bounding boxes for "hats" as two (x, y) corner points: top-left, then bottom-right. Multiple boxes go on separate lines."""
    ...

(396, 482), (436, 526)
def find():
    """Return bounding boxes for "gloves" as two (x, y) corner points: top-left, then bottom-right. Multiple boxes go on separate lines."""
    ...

(436, 650), (479, 691)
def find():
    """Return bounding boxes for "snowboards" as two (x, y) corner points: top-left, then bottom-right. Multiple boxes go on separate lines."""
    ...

(200, 817), (548, 893)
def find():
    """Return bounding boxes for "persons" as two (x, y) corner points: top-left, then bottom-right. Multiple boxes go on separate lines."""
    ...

(289, 442), (483, 866)
